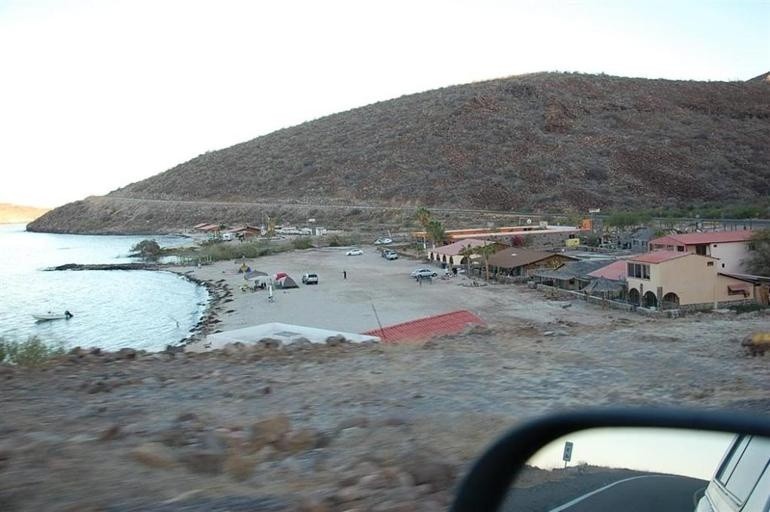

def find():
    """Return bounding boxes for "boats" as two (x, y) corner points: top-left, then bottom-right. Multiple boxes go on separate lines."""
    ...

(33, 312), (70, 320)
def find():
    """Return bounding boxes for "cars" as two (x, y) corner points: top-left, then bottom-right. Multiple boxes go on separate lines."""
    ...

(302, 273), (318, 284)
(346, 249), (362, 255)
(382, 249), (397, 259)
(412, 268), (437, 279)
(374, 238), (392, 245)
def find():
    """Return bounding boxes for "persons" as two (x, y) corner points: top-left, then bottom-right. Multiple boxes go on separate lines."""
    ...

(418, 274), (422, 287)
(446, 264), (450, 275)
(451, 267), (456, 276)
(344, 270), (348, 280)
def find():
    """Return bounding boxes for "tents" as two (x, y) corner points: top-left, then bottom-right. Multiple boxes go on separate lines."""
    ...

(243, 268), (298, 289)
